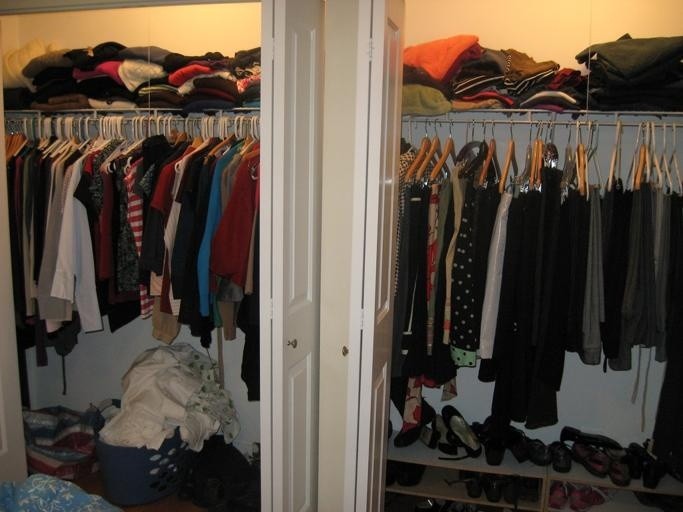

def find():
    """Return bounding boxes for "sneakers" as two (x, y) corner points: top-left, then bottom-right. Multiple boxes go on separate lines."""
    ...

(385, 399), (683, 512)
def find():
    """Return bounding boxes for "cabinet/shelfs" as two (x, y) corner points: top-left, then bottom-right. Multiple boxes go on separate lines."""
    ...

(541, 452), (682, 512)
(381, 429), (546, 511)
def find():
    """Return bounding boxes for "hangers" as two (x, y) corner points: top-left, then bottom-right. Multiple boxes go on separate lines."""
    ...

(401, 117), (682, 197)
(4, 113), (260, 172)
